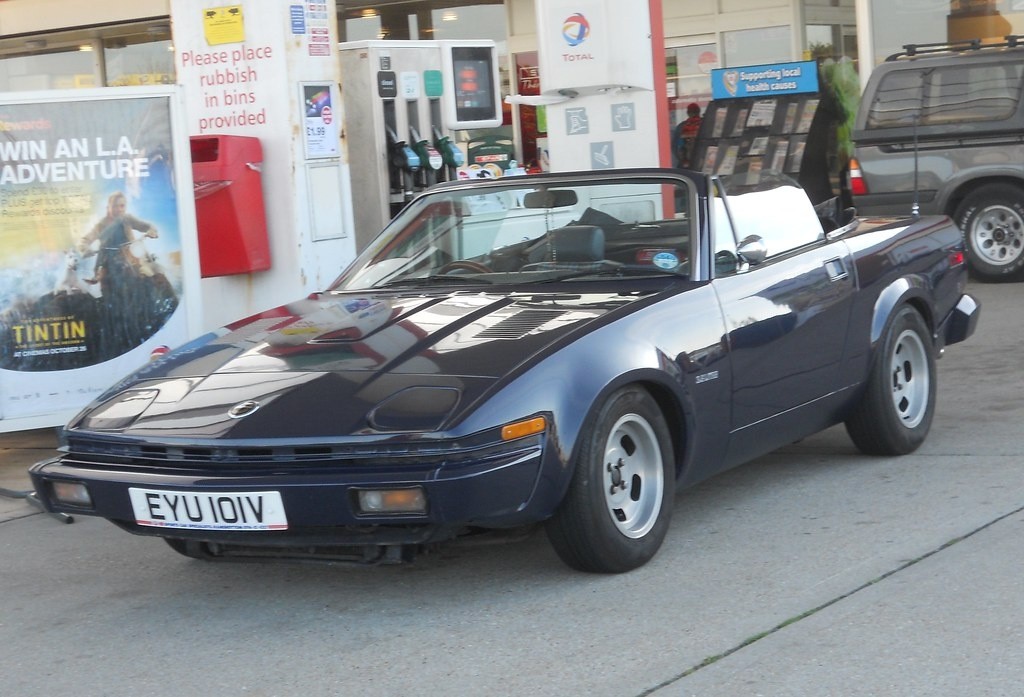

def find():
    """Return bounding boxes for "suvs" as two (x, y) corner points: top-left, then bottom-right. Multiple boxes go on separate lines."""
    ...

(839, 37), (1024, 280)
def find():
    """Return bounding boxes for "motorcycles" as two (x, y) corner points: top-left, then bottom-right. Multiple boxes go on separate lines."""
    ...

(79, 233), (161, 348)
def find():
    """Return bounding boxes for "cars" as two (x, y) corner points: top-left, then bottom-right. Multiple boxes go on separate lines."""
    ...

(22, 166), (986, 576)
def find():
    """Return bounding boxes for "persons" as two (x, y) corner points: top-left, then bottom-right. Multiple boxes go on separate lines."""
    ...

(79, 192), (159, 285)
(672, 103), (703, 169)
(675, 352), (709, 384)
